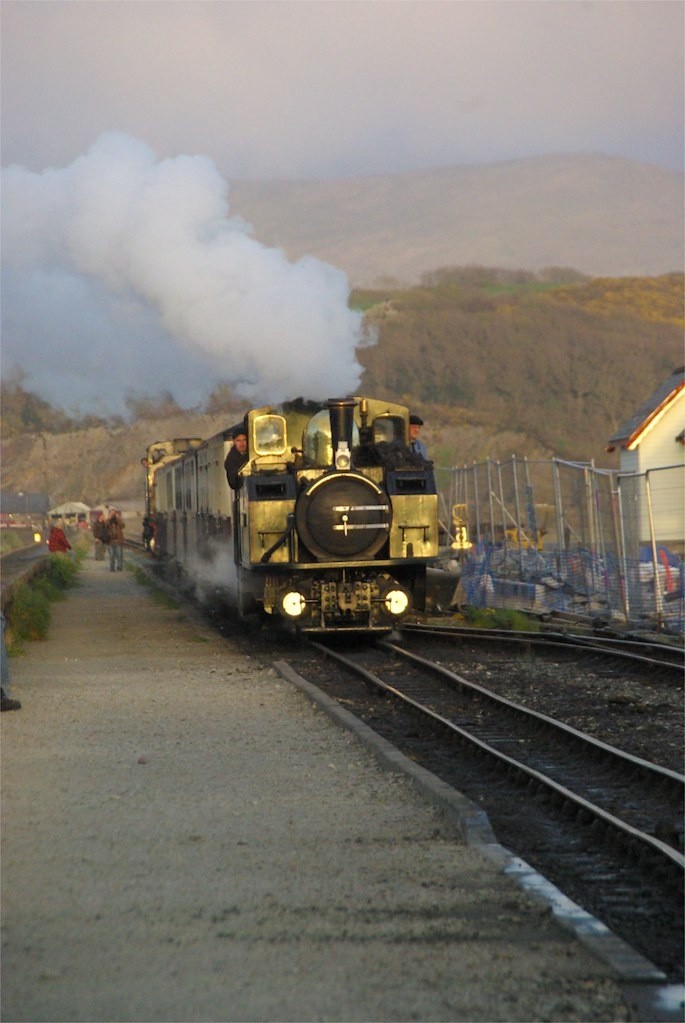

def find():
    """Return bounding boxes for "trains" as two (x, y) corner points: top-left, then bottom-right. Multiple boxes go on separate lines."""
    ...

(141, 394), (441, 639)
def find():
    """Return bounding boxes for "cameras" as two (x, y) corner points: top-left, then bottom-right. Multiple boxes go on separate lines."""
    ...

(112, 515), (116, 520)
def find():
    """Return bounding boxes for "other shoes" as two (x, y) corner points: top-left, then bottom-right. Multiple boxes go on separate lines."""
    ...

(0, 688), (21, 712)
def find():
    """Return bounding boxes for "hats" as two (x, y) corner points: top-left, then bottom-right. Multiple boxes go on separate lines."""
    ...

(233, 427), (247, 439)
(409, 416), (423, 425)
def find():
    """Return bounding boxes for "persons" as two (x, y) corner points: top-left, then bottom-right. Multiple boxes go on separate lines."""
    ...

(49, 521), (75, 555)
(94, 510), (125, 572)
(0, 613), (21, 713)
(409, 415), (427, 462)
(222, 429), (248, 490)
(140, 458), (148, 467)
